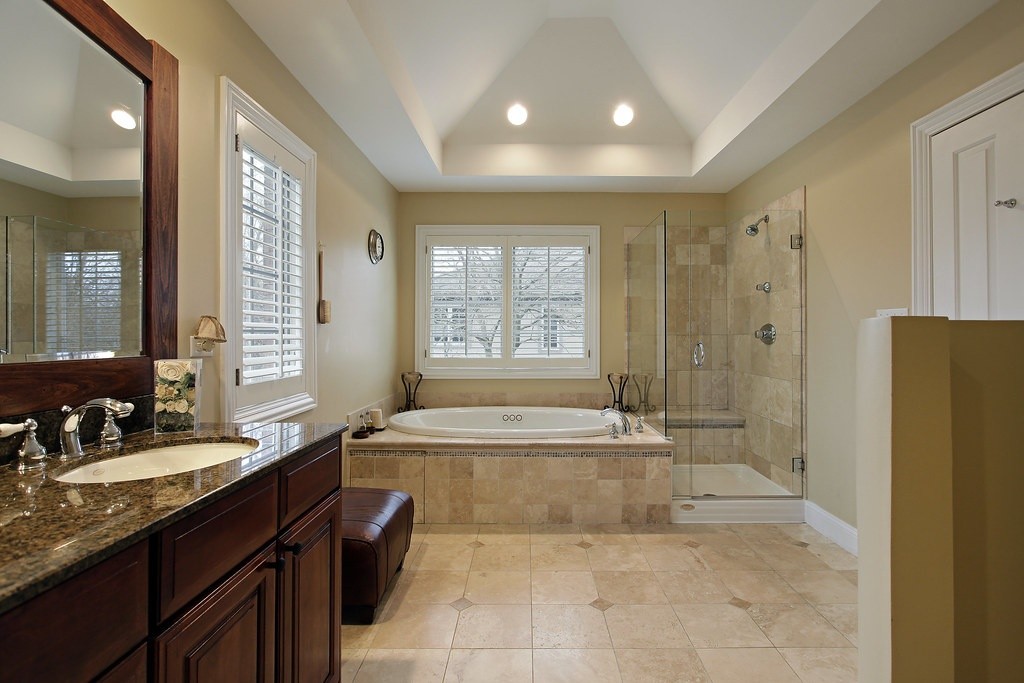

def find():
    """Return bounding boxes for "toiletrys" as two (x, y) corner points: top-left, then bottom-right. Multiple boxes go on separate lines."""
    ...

(358, 411), (373, 437)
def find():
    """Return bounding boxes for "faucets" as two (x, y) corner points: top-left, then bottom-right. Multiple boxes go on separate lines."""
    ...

(600, 407), (632, 436)
(58, 397), (130, 459)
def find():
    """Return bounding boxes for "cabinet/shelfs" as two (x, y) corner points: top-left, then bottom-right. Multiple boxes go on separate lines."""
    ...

(1, 427), (340, 683)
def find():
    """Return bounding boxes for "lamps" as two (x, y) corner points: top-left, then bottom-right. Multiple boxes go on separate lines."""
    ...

(506, 103), (529, 126)
(611, 100), (634, 127)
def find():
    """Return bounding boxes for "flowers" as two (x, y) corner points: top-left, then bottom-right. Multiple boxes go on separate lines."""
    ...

(152, 358), (203, 444)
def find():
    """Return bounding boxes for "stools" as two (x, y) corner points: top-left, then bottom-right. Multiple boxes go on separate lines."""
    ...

(339, 486), (415, 625)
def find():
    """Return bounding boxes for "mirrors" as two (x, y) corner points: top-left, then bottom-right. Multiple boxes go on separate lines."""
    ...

(0, 0), (152, 369)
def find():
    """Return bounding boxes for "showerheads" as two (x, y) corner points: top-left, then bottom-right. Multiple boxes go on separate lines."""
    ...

(746, 215), (769, 237)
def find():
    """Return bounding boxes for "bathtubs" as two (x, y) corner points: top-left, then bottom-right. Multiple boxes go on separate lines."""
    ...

(386, 406), (630, 440)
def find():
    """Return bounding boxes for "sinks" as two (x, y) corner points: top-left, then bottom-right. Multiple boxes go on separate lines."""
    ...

(47, 436), (259, 483)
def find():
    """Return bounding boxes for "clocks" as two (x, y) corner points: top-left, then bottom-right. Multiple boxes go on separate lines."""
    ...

(368, 228), (385, 265)
